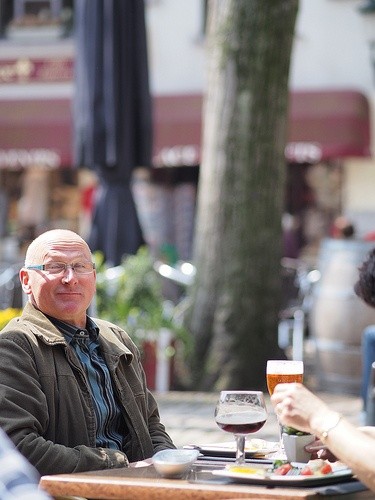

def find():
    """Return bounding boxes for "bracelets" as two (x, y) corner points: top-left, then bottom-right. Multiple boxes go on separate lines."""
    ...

(319, 414), (344, 441)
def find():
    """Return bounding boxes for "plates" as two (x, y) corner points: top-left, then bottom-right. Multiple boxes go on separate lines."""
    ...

(212, 462), (353, 480)
(200, 440), (279, 452)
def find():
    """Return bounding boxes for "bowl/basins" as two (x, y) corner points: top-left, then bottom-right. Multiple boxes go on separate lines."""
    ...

(153, 450), (198, 477)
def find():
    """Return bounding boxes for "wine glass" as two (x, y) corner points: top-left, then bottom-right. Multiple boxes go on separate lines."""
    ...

(267, 361), (303, 448)
(215, 391), (268, 464)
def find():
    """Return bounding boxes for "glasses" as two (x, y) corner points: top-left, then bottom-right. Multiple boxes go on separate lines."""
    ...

(26, 261), (95, 274)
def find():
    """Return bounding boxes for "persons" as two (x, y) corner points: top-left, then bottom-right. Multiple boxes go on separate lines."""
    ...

(272, 248), (375, 498)
(0, 230), (177, 475)
